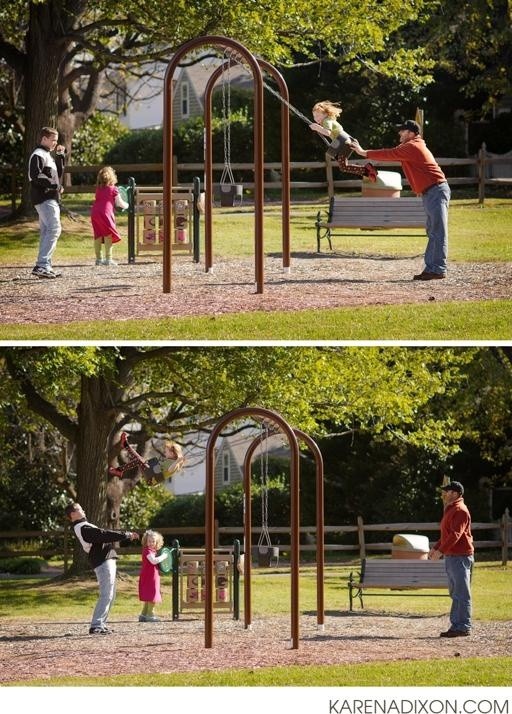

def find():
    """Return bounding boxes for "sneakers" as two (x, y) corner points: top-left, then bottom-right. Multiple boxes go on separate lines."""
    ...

(90, 627), (114, 635)
(95, 259), (117, 265)
(139, 614), (162, 622)
(441, 630), (470, 637)
(31, 266), (62, 279)
(414, 271), (447, 280)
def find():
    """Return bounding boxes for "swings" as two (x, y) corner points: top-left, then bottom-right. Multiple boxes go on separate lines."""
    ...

(141, 432), (258, 485)
(219, 54), (243, 207)
(223, 49), (355, 160)
(258, 422), (279, 568)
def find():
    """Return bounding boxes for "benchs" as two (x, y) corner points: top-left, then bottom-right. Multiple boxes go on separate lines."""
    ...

(348, 558), (451, 611)
(315, 196), (427, 253)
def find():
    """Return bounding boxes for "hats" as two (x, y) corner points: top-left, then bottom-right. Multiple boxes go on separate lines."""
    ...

(395, 120), (420, 132)
(440, 481), (464, 491)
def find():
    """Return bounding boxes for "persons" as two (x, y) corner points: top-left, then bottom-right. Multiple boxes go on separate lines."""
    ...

(350, 119), (451, 280)
(309, 99), (378, 184)
(29, 128), (66, 278)
(428, 481), (475, 638)
(91, 166), (129, 266)
(138, 530), (168, 622)
(109, 432), (184, 487)
(65, 502), (139, 635)
